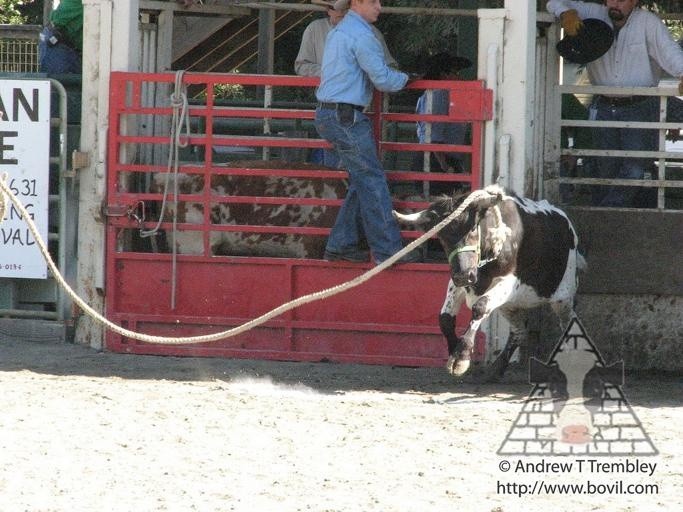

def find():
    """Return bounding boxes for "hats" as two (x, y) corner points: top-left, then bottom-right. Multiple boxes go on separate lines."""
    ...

(421, 51), (473, 80)
(556, 17), (614, 65)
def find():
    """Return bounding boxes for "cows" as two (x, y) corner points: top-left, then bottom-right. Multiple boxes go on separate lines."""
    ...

(390, 184), (588, 383)
(144, 159), (436, 261)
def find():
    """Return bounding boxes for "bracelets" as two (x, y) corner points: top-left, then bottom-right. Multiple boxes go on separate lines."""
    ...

(559, 10), (576, 18)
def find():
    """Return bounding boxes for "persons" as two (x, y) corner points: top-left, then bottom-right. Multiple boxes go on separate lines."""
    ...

(312, 0), (420, 268)
(413, 51), (471, 199)
(295, 1), (398, 171)
(38, 0), (193, 126)
(544, 0), (681, 208)
(560, 92), (589, 199)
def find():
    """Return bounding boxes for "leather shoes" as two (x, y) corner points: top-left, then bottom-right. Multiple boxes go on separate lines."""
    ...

(374, 251), (422, 269)
(324, 250), (370, 263)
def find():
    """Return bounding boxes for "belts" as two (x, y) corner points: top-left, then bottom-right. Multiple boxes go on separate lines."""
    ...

(46, 22), (82, 57)
(598, 95), (644, 106)
(317, 102), (363, 113)
